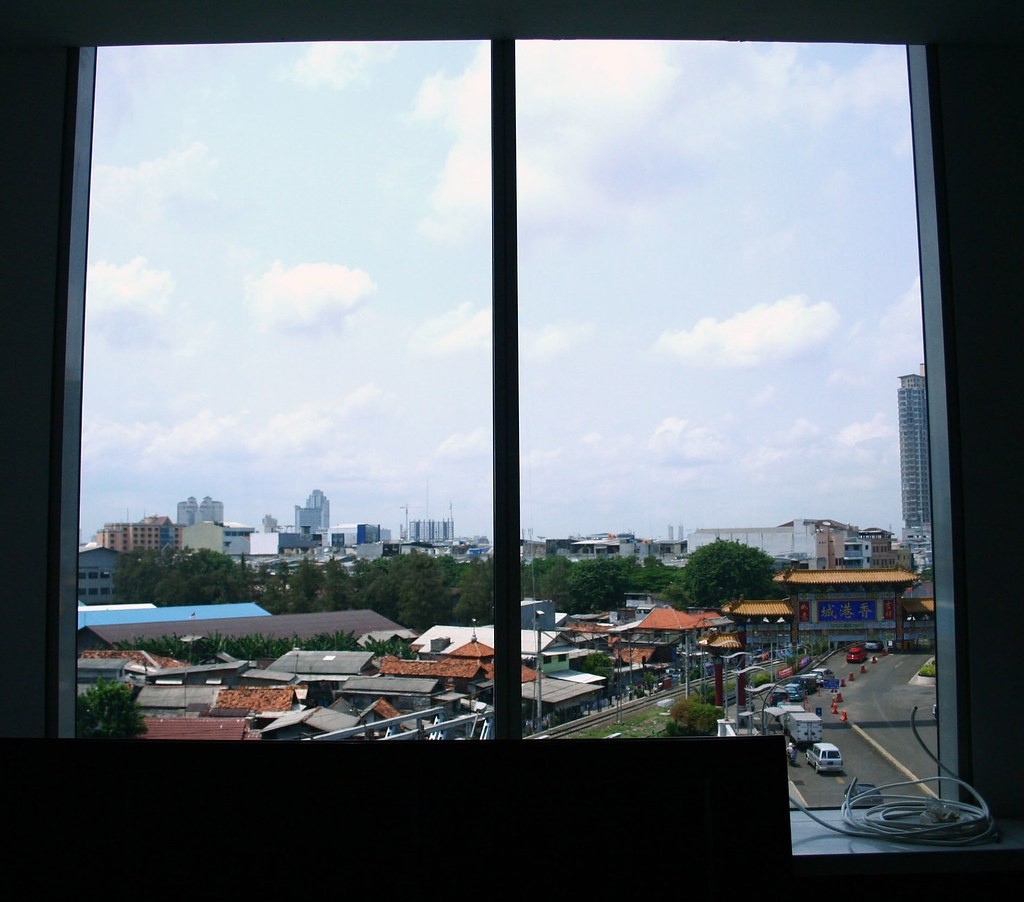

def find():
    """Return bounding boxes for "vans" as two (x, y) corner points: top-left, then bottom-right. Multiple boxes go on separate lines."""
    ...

(806, 743), (843, 775)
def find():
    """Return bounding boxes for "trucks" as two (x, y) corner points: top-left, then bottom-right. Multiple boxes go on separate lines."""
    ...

(785, 712), (823, 748)
(776, 700), (806, 733)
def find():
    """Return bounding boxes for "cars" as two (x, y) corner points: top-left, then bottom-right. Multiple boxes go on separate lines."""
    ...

(783, 682), (808, 703)
(811, 667), (834, 687)
(768, 688), (790, 708)
(799, 673), (825, 695)
(865, 639), (880, 652)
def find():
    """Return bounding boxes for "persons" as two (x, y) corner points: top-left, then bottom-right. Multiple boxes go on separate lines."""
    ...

(746, 702), (755, 711)
(786, 743), (797, 760)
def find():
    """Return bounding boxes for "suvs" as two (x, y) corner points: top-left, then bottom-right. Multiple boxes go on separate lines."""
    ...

(846, 647), (869, 664)
